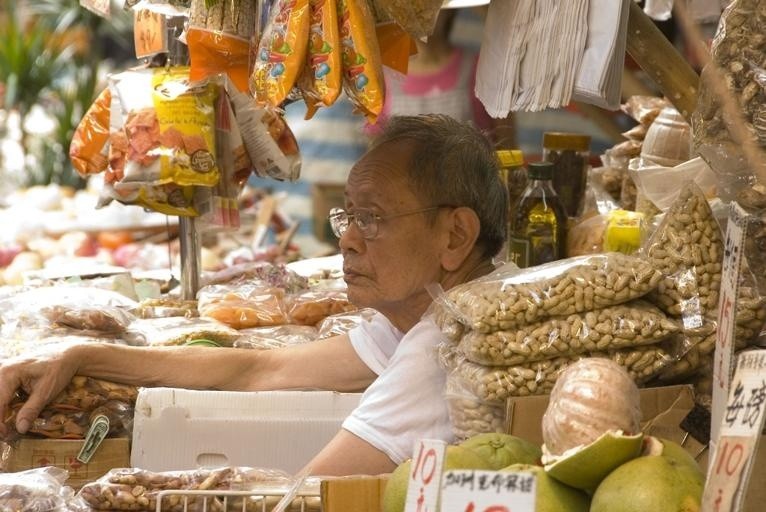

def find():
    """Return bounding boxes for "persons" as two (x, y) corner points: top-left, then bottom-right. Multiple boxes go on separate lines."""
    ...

(0, 111), (522, 483)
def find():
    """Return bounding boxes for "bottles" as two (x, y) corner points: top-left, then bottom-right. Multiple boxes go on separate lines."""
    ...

(494, 149), (566, 267)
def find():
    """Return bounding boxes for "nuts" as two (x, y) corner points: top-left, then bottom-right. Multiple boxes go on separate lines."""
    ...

(80, 467), (321, 512)
(9, 376), (139, 439)
(434, 185), (766, 443)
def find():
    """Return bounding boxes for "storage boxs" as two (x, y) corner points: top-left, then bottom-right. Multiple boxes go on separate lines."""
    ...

(0, 436), (132, 496)
(129, 387), (363, 480)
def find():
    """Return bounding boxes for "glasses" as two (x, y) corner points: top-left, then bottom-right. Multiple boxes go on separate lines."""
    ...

(327, 204), (457, 240)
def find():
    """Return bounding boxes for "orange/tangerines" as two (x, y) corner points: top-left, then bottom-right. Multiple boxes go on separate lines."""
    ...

(383, 358), (706, 512)
